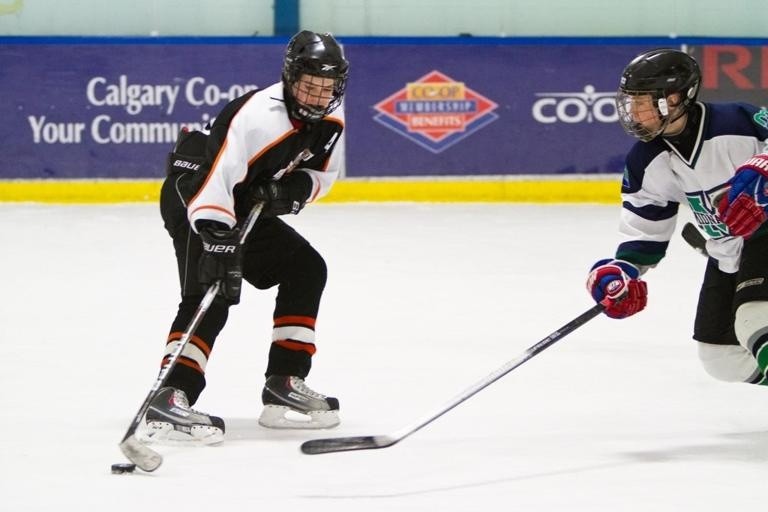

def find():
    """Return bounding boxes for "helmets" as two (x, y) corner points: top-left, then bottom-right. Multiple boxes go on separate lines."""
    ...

(617, 47), (702, 143)
(282, 29), (350, 123)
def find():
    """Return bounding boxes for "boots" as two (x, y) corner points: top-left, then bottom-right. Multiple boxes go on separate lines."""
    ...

(145, 386), (225, 435)
(262, 376), (340, 414)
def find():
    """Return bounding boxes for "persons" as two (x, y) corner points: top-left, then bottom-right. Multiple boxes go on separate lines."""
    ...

(586, 49), (768, 386)
(146, 30), (349, 441)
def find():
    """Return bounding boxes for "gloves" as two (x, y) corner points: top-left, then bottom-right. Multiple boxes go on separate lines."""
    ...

(585, 257), (648, 319)
(253, 178), (305, 217)
(717, 153), (768, 239)
(198, 226), (242, 305)
